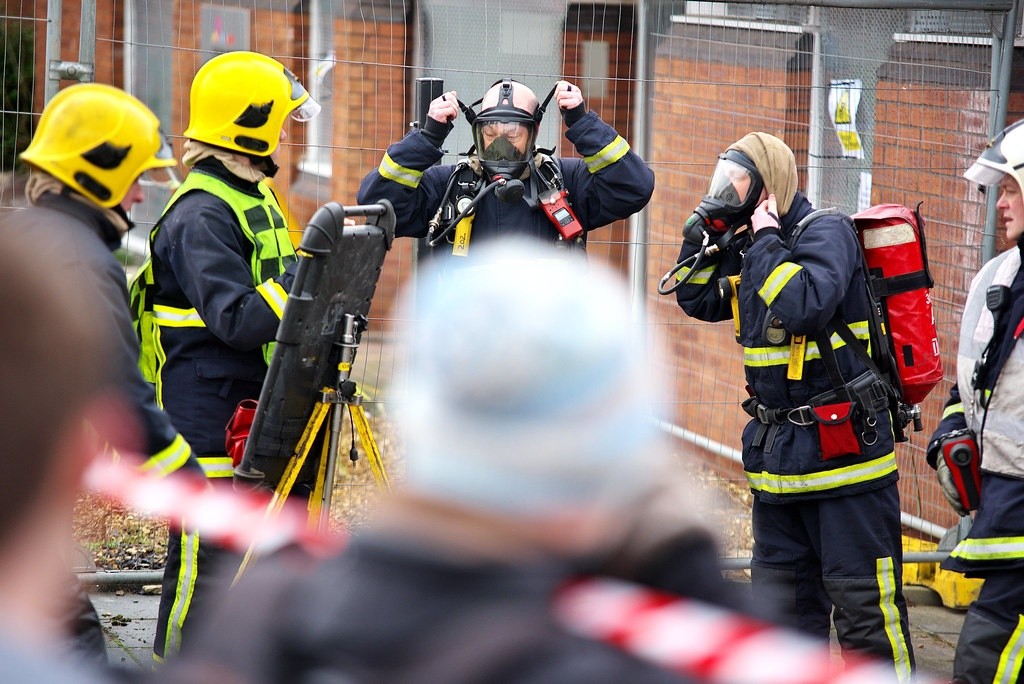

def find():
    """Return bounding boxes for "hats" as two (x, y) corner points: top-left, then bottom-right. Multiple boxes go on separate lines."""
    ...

(391, 238), (667, 518)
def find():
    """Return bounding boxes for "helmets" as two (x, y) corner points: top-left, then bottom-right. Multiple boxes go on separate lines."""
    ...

(20, 83), (178, 209)
(976, 118), (1024, 217)
(184, 50), (313, 158)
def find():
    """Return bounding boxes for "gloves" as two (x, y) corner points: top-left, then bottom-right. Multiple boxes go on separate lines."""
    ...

(933, 450), (970, 517)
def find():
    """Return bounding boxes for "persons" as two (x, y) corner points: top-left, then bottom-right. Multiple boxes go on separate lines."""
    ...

(0, 206), (156, 684)
(154, 238), (750, 684)
(926, 119), (1024, 684)
(356, 80), (655, 278)
(676, 131), (917, 684)
(5, 82), (218, 539)
(148, 52), (355, 666)
